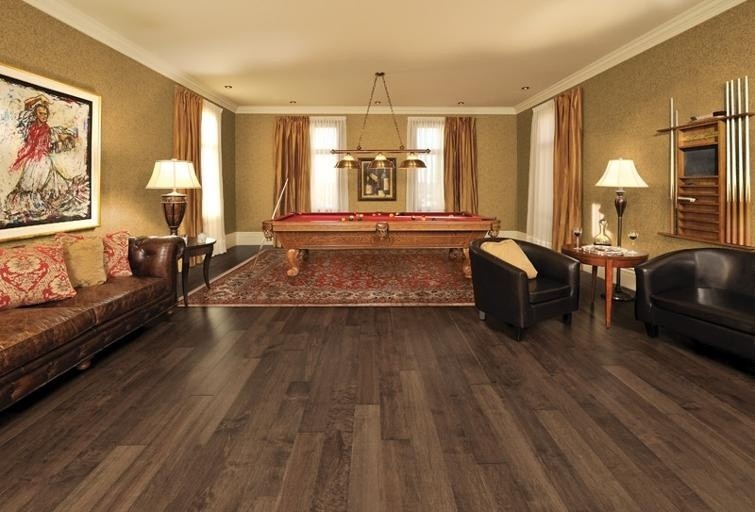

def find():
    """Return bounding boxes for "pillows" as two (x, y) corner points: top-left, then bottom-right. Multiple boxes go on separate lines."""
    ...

(98, 231), (133, 277)
(480, 238), (538, 280)
(54, 237), (107, 288)
(54, 232), (86, 241)
(0, 239), (78, 310)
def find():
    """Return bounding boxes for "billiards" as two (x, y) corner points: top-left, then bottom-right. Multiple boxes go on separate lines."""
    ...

(341, 217), (345, 221)
(422, 218), (425, 220)
(372, 213), (381, 216)
(432, 218), (436, 221)
(396, 213), (400, 216)
(423, 214), (425, 216)
(389, 214), (394, 218)
(356, 213), (363, 221)
(350, 216), (354, 220)
(412, 216), (415, 220)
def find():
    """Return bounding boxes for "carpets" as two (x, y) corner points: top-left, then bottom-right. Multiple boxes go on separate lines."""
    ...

(177, 249), (477, 311)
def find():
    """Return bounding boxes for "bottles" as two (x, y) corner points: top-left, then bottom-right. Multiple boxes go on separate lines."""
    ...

(594, 220), (612, 250)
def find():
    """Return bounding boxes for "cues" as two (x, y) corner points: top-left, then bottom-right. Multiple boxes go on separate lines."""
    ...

(251, 177), (290, 271)
(725, 77), (751, 247)
(666, 97), (680, 235)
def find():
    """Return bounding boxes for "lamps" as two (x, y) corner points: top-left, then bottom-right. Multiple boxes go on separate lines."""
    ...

(594, 158), (650, 304)
(144, 157), (202, 235)
(327, 71), (431, 171)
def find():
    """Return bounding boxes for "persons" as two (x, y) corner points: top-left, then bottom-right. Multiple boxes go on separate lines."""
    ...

(3, 95), (90, 223)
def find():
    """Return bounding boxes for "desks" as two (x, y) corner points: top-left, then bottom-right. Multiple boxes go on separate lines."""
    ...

(261, 209), (503, 282)
(180, 237), (217, 308)
(561, 244), (650, 330)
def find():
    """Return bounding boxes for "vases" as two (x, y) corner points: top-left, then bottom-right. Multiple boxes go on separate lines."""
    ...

(591, 219), (612, 247)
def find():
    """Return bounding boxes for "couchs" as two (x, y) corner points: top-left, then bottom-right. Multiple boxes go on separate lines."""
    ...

(0, 236), (187, 411)
(635, 248), (754, 380)
(467, 235), (584, 342)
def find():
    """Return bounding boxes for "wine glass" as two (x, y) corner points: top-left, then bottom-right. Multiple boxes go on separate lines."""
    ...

(572, 226), (583, 250)
(628, 231), (639, 256)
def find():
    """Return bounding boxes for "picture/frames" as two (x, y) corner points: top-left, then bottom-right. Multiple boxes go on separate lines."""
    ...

(356, 157), (397, 202)
(0, 62), (103, 242)
